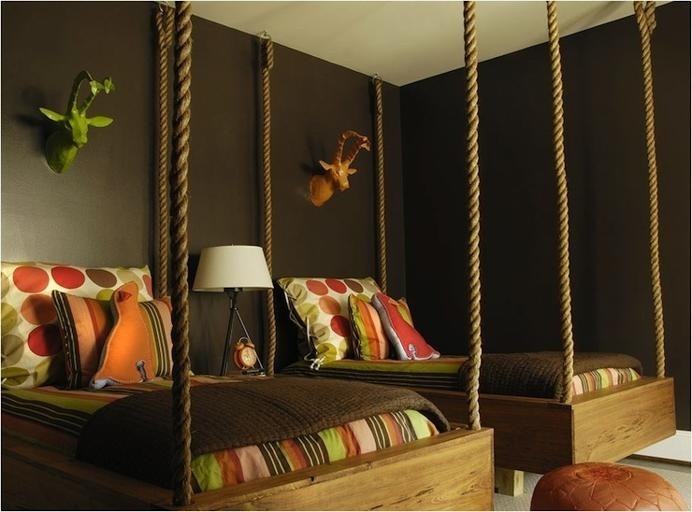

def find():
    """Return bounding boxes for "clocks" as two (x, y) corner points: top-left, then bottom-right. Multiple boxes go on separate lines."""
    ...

(232, 336), (258, 372)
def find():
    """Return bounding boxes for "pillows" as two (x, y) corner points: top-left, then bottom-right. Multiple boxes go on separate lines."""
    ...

(2, 260), (173, 391)
(277, 276), (440, 371)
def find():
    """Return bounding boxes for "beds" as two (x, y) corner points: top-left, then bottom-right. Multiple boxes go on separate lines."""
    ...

(277, 350), (676, 498)
(2, 377), (495, 512)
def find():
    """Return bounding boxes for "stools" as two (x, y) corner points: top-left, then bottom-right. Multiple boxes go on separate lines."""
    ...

(529, 462), (688, 511)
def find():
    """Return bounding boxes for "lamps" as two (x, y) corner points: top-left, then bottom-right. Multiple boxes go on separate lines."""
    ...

(192, 243), (275, 376)
